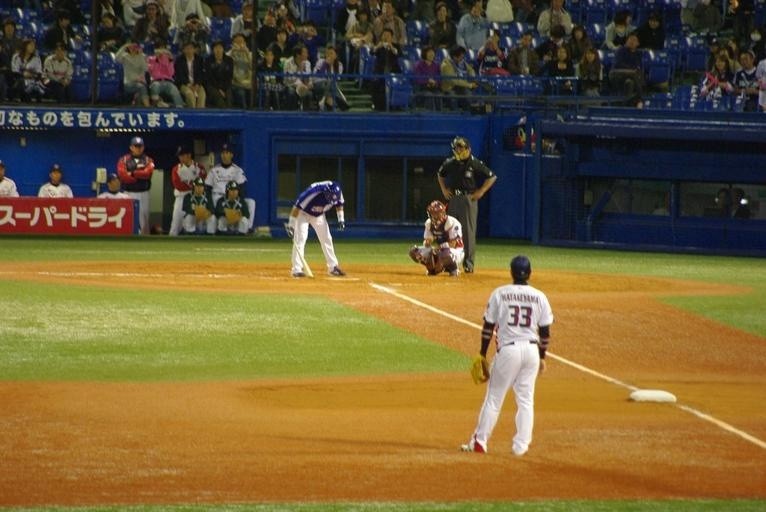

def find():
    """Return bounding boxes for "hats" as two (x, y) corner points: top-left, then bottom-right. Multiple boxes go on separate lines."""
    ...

(192, 176), (206, 187)
(175, 144), (190, 156)
(131, 137), (144, 145)
(107, 172), (117, 183)
(0, 159), (5, 167)
(219, 144), (233, 152)
(511, 255), (530, 280)
(49, 163), (62, 173)
(225, 181), (240, 192)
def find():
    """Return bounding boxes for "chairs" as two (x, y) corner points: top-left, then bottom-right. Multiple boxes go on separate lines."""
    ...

(0, 0), (766, 112)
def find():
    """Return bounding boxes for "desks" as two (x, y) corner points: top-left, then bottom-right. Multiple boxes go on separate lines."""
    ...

(1, 194), (141, 241)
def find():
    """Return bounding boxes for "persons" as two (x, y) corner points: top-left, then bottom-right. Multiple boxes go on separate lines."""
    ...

(116, 137), (154, 235)
(37, 164), (74, 198)
(460, 255), (554, 456)
(436, 135), (499, 274)
(97, 173), (127, 198)
(344, 0), (765, 116)
(1, 163), (20, 196)
(408, 200), (466, 277)
(653, 187), (751, 220)
(169, 143), (251, 237)
(0, 0), (352, 112)
(283, 180), (347, 277)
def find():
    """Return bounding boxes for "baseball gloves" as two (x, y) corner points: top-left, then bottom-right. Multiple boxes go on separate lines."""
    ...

(410, 246), (429, 264)
(471, 357), (490, 384)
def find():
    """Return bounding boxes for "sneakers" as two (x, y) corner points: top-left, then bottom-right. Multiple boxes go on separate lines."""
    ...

(460, 444), (487, 453)
(328, 266), (344, 275)
(427, 262), (474, 276)
(292, 272), (305, 277)
(512, 445), (526, 456)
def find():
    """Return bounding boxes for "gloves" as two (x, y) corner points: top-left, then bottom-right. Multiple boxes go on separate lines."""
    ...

(288, 227), (295, 238)
(337, 221), (344, 231)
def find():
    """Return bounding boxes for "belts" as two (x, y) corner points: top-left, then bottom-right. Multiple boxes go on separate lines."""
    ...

(155, 78), (172, 82)
(450, 190), (474, 195)
(508, 341), (537, 345)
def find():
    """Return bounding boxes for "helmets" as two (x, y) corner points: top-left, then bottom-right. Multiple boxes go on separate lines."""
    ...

(427, 200), (447, 230)
(324, 182), (342, 206)
(450, 136), (471, 160)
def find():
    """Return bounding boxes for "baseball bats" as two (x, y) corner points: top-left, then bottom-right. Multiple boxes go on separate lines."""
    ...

(289, 238), (312, 276)
